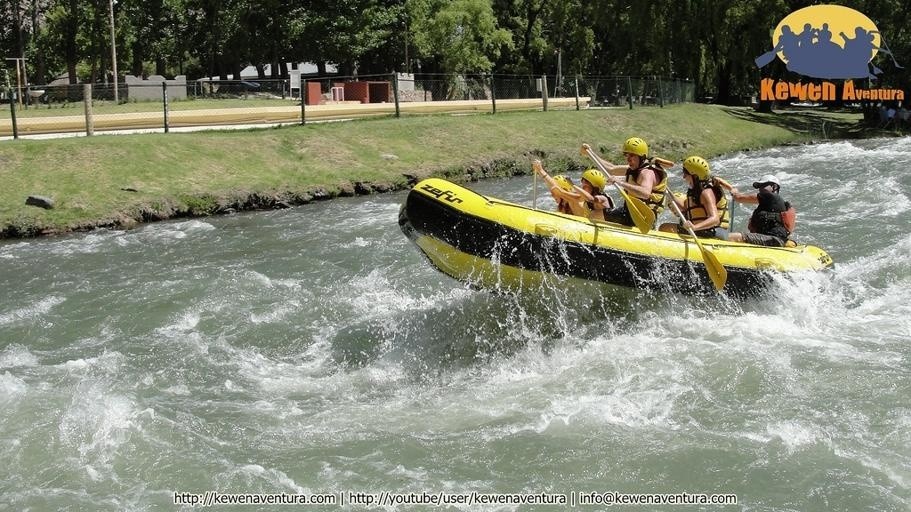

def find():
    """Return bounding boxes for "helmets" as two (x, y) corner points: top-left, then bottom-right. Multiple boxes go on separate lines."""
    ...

(582, 169), (605, 194)
(552, 175), (574, 192)
(623, 137), (648, 157)
(684, 156), (709, 180)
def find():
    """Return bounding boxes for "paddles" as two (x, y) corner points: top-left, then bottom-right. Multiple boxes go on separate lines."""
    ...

(586, 148), (655, 235)
(666, 191), (727, 291)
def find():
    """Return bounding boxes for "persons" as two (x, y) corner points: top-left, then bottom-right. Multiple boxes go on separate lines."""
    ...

(725, 174), (796, 246)
(551, 168), (616, 221)
(579, 136), (674, 226)
(657, 155), (730, 240)
(533, 157), (584, 217)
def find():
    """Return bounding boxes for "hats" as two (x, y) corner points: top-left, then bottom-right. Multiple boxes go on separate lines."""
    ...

(753, 175), (780, 189)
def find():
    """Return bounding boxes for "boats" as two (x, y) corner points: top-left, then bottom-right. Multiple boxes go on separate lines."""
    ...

(400, 177), (836, 310)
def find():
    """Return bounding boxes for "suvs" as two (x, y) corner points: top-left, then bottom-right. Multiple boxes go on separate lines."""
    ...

(28, 86), (47, 98)
(40, 87), (83, 103)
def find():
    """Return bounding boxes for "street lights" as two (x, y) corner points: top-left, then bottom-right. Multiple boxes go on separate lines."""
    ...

(108, 1), (120, 101)
(402, 21), (409, 72)
(1, 69), (11, 90)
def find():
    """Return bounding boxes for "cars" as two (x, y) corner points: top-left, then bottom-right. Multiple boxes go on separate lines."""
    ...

(217, 82), (260, 97)
(187, 81), (217, 96)
(250, 82), (261, 90)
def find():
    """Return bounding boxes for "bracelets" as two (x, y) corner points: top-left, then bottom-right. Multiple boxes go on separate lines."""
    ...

(540, 174), (549, 180)
